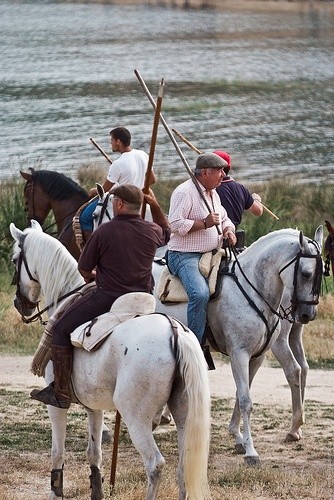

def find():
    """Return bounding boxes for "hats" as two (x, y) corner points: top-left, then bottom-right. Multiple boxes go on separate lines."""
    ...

(109, 184), (142, 204)
(212, 151), (231, 166)
(196, 152), (228, 168)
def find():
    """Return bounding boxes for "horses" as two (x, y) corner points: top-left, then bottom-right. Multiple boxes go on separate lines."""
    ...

(149, 227), (326, 466)
(9, 218), (214, 500)
(20, 167), (100, 262)
(310, 220), (334, 277)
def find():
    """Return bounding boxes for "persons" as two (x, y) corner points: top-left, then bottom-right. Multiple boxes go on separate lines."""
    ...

(214, 150), (263, 234)
(165, 153), (238, 342)
(30, 184), (171, 408)
(79, 128), (156, 245)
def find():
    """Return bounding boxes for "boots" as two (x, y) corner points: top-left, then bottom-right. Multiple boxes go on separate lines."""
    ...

(30, 344), (74, 409)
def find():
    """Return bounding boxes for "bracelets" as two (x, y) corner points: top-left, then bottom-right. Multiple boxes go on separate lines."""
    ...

(202, 219), (207, 229)
(253, 197), (260, 202)
